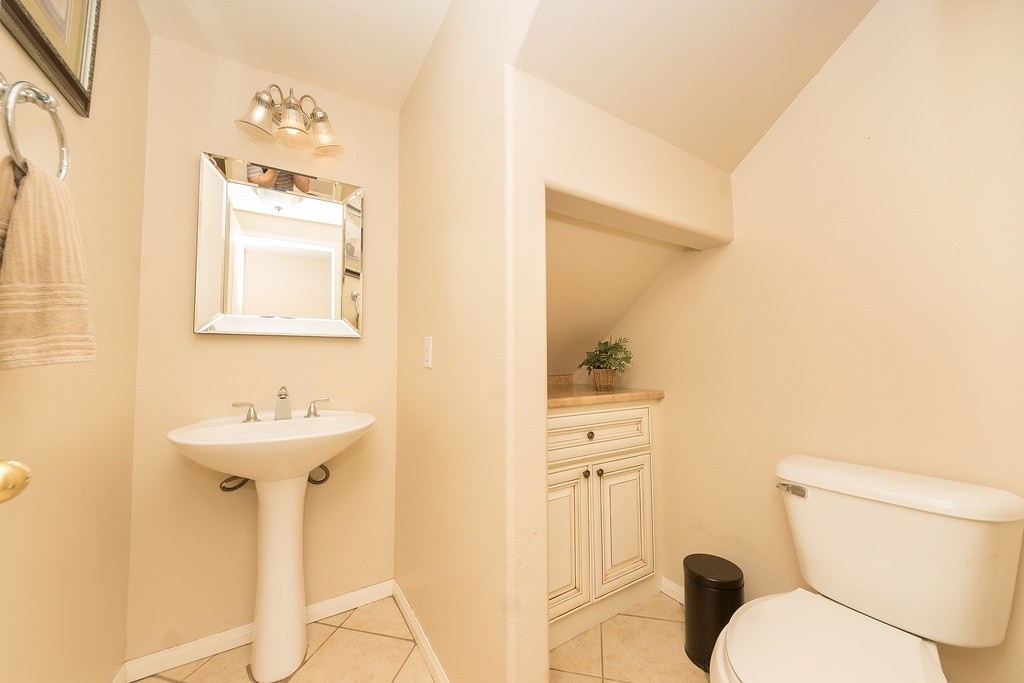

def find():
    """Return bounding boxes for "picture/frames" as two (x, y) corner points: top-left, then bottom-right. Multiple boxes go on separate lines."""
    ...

(0, 0), (102, 120)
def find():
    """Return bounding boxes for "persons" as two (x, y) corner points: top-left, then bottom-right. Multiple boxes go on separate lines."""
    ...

(246, 161), (310, 196)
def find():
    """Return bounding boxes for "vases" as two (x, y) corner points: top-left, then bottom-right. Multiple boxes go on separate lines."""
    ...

(593, 367), (616, 392)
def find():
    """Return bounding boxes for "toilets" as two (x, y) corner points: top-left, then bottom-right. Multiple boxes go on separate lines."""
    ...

(709, 454), (1024, 683)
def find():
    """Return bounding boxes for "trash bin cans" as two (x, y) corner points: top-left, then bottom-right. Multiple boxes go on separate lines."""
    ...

(682, 553), (745, 672)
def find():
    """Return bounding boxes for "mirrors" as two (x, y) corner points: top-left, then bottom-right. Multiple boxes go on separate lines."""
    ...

(192, 151), (365, 340)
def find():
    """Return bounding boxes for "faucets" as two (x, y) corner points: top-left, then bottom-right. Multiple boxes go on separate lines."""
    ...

(274, 386), (292, 419)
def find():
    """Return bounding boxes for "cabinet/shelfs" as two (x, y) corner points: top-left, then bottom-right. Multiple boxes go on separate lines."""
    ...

(548, 401), (659, 651)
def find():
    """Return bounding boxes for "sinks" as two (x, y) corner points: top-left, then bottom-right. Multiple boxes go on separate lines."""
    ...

(166, 415), (375, 481)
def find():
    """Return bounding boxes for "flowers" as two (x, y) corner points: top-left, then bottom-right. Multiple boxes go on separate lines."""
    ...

(579, 335), (637, 376)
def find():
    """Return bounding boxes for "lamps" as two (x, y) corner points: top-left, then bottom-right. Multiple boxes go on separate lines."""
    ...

(235, 84), (343, 158)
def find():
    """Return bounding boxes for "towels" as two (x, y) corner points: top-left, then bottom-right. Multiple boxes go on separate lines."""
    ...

(0, 155), (96, 370)
